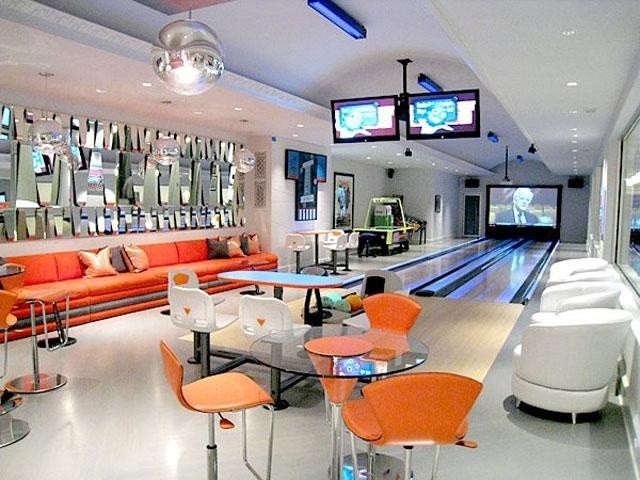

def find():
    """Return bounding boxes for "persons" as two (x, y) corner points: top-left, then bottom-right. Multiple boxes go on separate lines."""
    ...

(494, 185), (540, 227)
(338, 184), (351, 218)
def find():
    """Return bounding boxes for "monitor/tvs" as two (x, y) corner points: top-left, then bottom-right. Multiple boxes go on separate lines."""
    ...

(331, 95), (400, 143)
(485, 184), (563, 240)
(406, 88), (481, 140)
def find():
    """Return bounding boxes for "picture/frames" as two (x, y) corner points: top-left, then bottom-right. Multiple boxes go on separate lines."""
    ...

(285, 148), (327, 221)
(333, 172), (354, 232)
(435, 195), (441, 213)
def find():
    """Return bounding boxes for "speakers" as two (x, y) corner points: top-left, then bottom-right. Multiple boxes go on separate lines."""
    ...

(568, 179), (584, 188)
(465, 179), (480, 188)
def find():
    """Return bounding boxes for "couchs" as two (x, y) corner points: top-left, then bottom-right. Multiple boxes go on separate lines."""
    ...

(0, 239), (278, 344)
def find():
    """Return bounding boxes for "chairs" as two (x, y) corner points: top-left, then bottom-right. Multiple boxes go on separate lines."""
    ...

(0, 262), (76, 450)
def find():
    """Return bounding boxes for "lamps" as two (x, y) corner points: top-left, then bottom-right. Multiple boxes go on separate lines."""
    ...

(417, 72), (443, 93)
(487, 132), (499, 144)
(306, 0), (367, 40)
